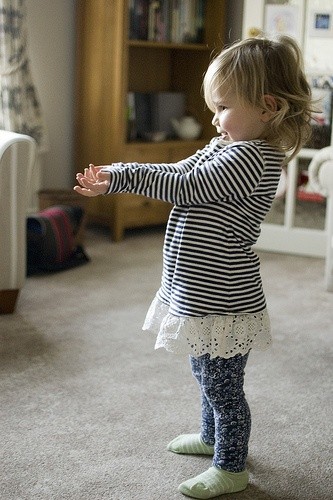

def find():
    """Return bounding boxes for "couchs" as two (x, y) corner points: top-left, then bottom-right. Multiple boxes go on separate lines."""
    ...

(307, 145), (333, 293)
(0, 129), (40, 315)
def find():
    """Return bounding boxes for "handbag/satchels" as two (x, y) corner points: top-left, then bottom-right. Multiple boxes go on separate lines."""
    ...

(26, 204), (92, 277)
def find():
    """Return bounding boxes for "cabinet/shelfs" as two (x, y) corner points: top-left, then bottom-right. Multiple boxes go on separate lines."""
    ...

(71, 0), (232, 242)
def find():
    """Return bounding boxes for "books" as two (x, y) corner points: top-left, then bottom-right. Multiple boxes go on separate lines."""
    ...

(129, 0), (205, 44)
(126, 90), (185, 143)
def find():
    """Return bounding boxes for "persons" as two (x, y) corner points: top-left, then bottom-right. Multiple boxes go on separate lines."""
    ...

(74, 35), (326, 500)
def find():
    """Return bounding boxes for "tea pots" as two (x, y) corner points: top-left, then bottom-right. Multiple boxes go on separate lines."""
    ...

(169, 116), (203, 140)
